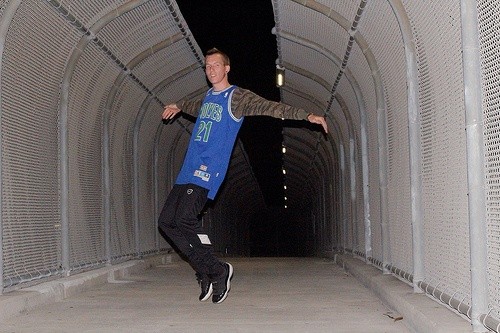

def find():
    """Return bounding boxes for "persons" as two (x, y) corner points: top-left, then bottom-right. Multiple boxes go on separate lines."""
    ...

(155, 48), (327, 303)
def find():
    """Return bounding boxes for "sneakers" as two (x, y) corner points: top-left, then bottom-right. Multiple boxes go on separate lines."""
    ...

(210, 261), (234, 304)
(195, 272), (213, 302)
